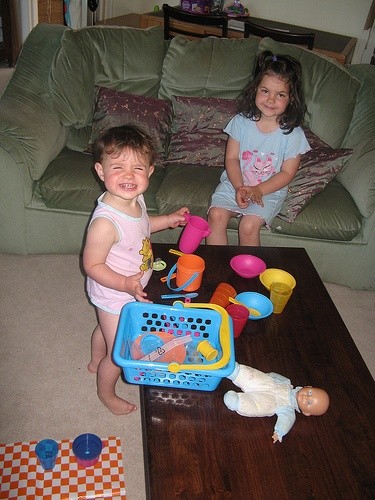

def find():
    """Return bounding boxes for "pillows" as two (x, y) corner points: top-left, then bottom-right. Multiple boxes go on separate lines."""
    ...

(164, 95), (242, 167)
(82, 85), (173, 169)
(275, 127), (354, 224)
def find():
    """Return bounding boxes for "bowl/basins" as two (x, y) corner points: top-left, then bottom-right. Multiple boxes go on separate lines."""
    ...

(260, 268), (296, 290)
(230, 254), (266, 278)
(235, 292), (274, 320)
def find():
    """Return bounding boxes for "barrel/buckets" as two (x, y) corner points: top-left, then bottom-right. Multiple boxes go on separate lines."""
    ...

(167, 255), (204, 292)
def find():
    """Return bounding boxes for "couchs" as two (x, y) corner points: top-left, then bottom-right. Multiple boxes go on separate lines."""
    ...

(0, 23), (375, 292)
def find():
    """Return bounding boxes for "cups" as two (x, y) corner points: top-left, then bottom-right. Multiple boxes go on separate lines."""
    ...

(178, 212), (211, 254)
(209, 283), (237, 311)
(270, 282), (292, 314)
(35, 439), (59, 470)
(72, 433), (102, 468)
(225, 305), (249, 338)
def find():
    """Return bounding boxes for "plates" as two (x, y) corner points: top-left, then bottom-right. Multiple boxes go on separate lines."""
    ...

(130, 331), (187, 365)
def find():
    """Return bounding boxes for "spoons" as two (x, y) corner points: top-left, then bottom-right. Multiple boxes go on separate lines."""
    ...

(228, 297), (261, 317)
(161, 293), (198, 299)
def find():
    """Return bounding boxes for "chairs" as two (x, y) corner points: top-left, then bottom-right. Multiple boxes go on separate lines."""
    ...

(162, 3), (229, 41)
(244, 21), (316, 51)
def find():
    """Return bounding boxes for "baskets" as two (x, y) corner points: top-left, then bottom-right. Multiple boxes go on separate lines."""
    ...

(113, 301), (235, 391)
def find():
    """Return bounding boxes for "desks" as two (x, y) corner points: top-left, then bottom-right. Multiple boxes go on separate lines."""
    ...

(138, 243), (375, 500)
(141, 5), (358, 66)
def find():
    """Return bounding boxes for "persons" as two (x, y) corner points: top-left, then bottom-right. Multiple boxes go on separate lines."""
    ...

(82, 124), (189, 415)
(223, 360), (329, 444)
(205, 50), (311, 247)
